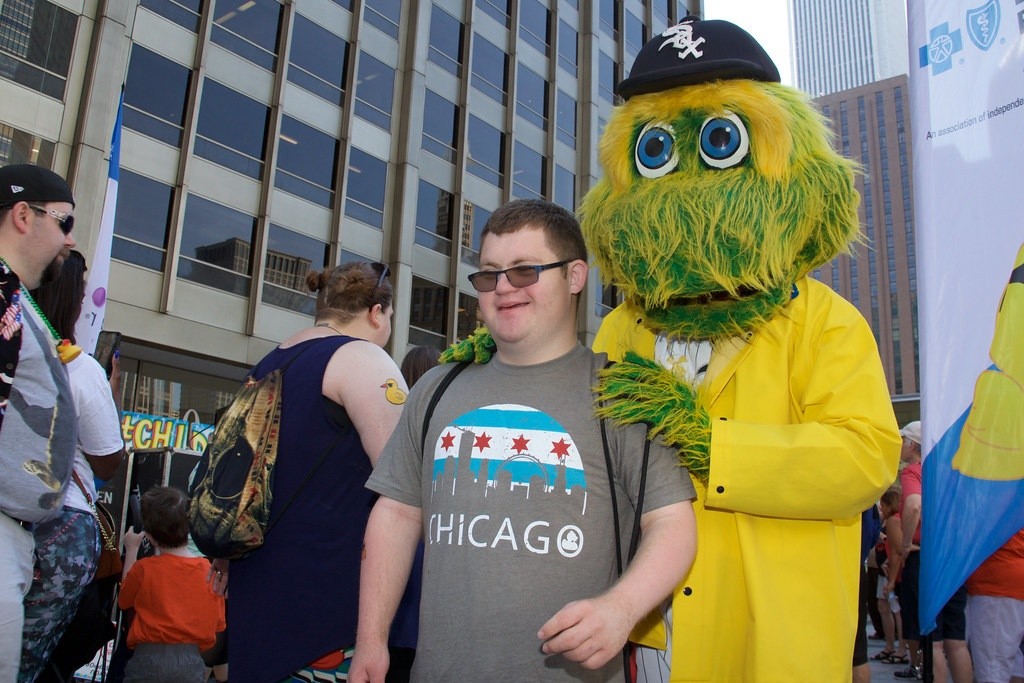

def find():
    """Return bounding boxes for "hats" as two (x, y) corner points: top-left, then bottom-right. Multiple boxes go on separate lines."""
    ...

(0, 164), (75, 211)
(899, 420), (922, 445)
(617, 15), (780, 102)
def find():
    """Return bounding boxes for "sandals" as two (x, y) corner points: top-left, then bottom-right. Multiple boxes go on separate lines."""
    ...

(869, 650), (895, 660)
(881, 654), (909, 664)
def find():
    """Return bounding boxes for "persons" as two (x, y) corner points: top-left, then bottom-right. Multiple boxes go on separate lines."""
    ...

(852, 419), (1024, 683)
(0, 164), (123, 683)
(400, 346), (443, 387)
(206, 262), (410, 683)
(347, 197), (696, 683)
(118, 487), (226, 683)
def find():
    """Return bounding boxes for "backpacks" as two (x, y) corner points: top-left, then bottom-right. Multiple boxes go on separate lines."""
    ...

(190, 334), (352, 562)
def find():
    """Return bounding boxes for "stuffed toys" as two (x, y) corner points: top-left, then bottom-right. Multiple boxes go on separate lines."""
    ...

(444, 18), (903, 682)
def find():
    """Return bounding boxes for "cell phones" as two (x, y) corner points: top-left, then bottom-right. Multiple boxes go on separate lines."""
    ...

(93, 330), (121, 381)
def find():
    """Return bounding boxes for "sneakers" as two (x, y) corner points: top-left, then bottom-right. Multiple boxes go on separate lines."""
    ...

(894, 665), (923, 680)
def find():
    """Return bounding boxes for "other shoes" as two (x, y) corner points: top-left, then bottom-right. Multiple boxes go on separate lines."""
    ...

(868, 633), (882, 639)
(884, 633), (898, 641)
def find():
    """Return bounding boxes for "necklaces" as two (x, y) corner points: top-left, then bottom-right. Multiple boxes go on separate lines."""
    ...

(1, 255), (65, 343)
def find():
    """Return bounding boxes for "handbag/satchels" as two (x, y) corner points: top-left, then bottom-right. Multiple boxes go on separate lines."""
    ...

(93, 501), (124, 576)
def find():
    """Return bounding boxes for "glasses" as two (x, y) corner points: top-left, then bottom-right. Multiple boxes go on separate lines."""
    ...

(467, 256), (582, 292)
(6, 203), (75, 236)
(369, 263), (391, 313)
